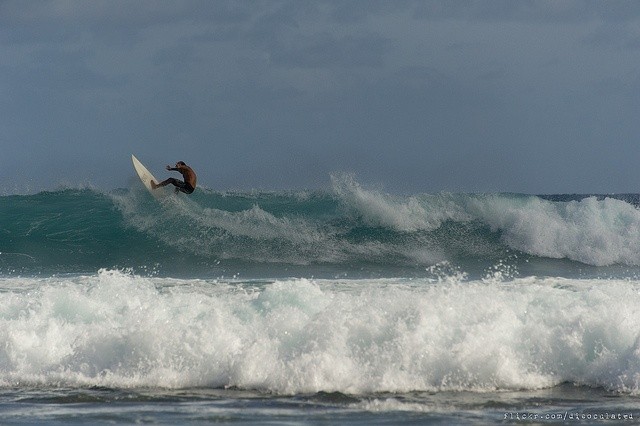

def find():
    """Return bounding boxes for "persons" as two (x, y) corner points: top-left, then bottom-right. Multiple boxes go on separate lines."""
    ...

(150, 160), (197, 196)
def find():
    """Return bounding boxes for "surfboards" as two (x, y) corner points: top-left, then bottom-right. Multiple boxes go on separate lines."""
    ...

(130, 152), (165, 200)
